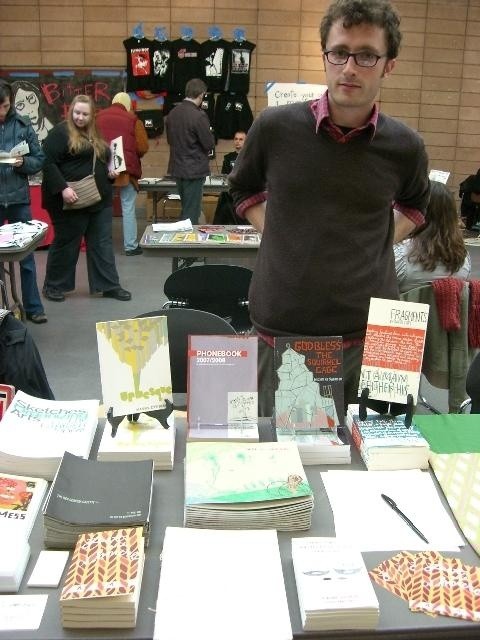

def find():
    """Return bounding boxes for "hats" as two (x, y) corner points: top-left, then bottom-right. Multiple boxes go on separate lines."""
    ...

(112, 92), (131, 112)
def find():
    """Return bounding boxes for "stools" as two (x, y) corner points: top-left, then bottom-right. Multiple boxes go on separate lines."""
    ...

(163, 195), (185, 218)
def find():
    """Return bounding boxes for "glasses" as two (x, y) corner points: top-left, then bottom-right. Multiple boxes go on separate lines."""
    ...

(324, 48), (387, 67)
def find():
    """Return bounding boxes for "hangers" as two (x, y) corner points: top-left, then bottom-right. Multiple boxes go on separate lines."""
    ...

(133, 31), (248, 45)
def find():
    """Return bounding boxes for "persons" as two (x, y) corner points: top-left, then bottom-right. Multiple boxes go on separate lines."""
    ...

(165, 78), (215, 224)
(221, 130), (246, 175)
(392, 181), (472, 293)
(226, 0), (432, 418)
(40, 95), (131, 301)
(0, 78), (47, 324)
(94, 91), (149, 256)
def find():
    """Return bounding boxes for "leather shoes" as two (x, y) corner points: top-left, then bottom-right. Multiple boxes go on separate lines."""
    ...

(103, 288), (131, 301)
(42, 280), (65, 301)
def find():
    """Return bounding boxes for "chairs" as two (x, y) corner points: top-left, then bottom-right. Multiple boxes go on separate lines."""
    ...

(401, 283), (472, 414)
(134, 309), (239, 394)
(163, 265), (254, 334)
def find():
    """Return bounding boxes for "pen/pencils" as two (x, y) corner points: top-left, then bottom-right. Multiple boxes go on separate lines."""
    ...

(383, 496), (429, 545)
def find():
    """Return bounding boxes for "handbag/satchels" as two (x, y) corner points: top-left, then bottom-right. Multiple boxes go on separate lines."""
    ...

(63, 175), (101, 211)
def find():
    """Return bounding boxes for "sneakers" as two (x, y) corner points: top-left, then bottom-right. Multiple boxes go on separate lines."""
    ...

(127, 246), (143, 256)
(26, 311), (47, 323)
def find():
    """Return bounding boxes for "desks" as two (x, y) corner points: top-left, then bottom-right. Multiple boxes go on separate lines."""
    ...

(0, 220), (47, 324)
(134, 174), (238, 224)
(140, 225), (267, 274)
(0, 413), (480, 640)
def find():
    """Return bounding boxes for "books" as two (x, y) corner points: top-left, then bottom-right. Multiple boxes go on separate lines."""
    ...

(187, 427), (260, 442)
(142, 218), (261, 245)
(0, 140), (29, 166)
(153, 526), (293, 639)
(291, 537), (380, 631)
(186, 333), (260, 426)
(354, 296), (430, 407)
(0, 384), (177, 628)
(272, 334), (346, 432)
(95, 316), (174, 417)
(182, 442), (314, 531)
(276, 414), (429, 471)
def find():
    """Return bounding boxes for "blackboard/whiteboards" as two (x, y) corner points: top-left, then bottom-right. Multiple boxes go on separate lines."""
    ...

(1, 67), (130, 122)
